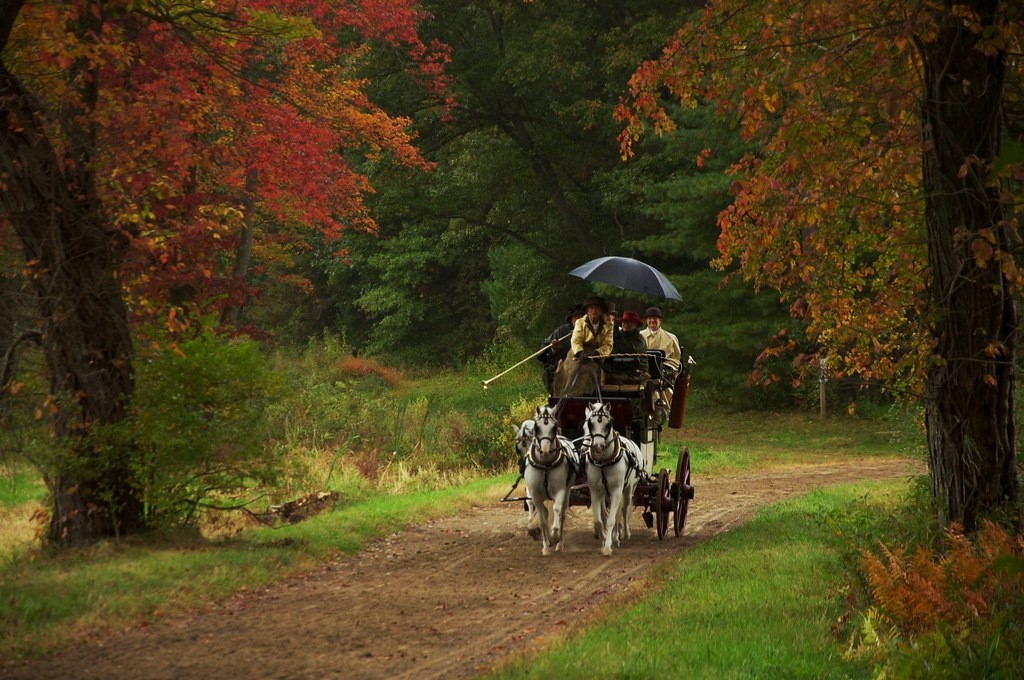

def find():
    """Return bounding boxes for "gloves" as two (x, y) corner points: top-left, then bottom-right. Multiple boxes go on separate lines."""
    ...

(578, 350), (589, 364)
(586, 348), (599, 361)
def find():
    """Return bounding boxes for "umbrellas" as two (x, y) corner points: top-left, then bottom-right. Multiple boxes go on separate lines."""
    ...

(568, 252), (684, 318)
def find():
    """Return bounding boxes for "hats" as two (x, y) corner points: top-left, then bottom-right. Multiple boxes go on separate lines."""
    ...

(565, 303), (585, 323)
(616, 311), (644, 328)
(643, 306), (663, 319)
(604, 302), (618, 317)
(581, 295), (608, 314)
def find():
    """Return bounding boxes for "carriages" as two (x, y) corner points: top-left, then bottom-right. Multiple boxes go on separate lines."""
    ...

(499, 349), (695, 558)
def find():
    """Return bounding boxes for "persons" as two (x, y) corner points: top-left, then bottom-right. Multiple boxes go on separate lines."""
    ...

(556, 296), (613, 397)
(537, 304), (585, 394)
(639, 308), (681, 409)
(605, 303), (650, 414)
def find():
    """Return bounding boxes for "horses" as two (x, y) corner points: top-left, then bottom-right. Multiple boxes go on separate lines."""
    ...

(512, 401), (645, 557)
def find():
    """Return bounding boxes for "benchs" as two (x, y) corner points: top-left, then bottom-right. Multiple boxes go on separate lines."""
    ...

(557, 356), (649, 392)
(647, 348), (666, 385)
